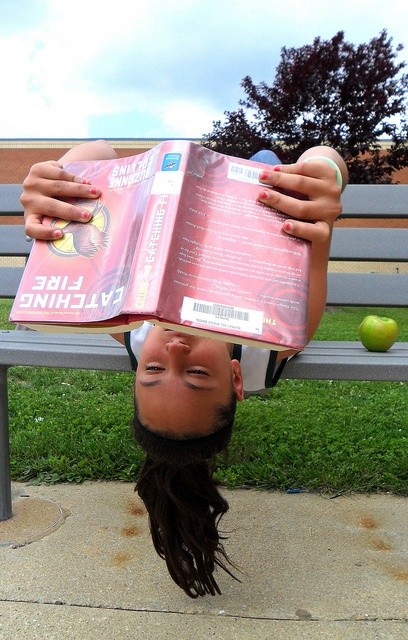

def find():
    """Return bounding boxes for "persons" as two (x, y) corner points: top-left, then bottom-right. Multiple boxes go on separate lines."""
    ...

(19, 139), (349, 599)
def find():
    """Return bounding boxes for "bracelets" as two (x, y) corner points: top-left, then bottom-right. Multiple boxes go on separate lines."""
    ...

(302, 155), (343, 191)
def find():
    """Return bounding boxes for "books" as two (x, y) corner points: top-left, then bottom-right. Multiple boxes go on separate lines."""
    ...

(7, 138), (313, 352)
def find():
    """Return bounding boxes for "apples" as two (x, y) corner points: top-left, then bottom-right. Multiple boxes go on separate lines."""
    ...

(358, 313), (399, 352)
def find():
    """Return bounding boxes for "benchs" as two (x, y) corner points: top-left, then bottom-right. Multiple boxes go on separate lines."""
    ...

(0, 175), (408, 523)
